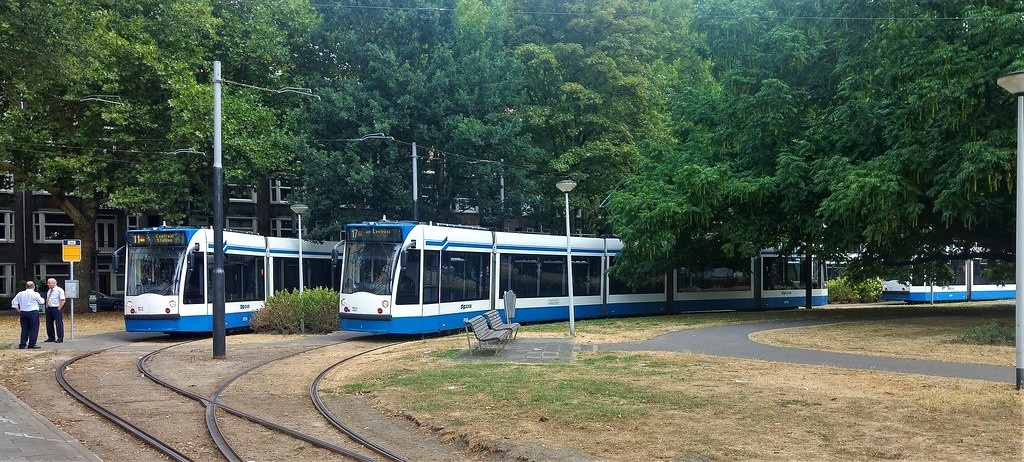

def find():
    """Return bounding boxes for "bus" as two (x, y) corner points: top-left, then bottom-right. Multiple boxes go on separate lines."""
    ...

(111, 219), (345, 339)
(879, 236), (1016, 304)
(336, 158), (830, 335)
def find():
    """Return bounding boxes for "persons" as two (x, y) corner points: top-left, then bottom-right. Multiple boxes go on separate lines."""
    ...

(44, 278), (65, 343)
(11, 281), (46, 349)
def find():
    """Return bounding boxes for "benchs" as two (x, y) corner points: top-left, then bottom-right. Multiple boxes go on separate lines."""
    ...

(465, 314), (509, 357)
(482, 310), (521, 343)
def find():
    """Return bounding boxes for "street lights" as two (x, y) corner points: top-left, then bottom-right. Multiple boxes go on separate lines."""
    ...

(289, 203), (309, 335)
(997, 69), (1024, 393)
(555, 178), (577, 336)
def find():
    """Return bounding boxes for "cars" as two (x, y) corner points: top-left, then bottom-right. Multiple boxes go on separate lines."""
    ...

(88, 289), (124, 312)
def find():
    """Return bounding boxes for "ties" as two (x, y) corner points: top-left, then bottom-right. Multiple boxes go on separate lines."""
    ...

(46, 289), (53, 308)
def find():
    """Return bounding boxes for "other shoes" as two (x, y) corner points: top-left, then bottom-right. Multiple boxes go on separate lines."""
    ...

(28, 345), (41, 349)
(55, 338), (63, 343)
(19, 345), (27, 349)
(44, 338), (55, 342)
